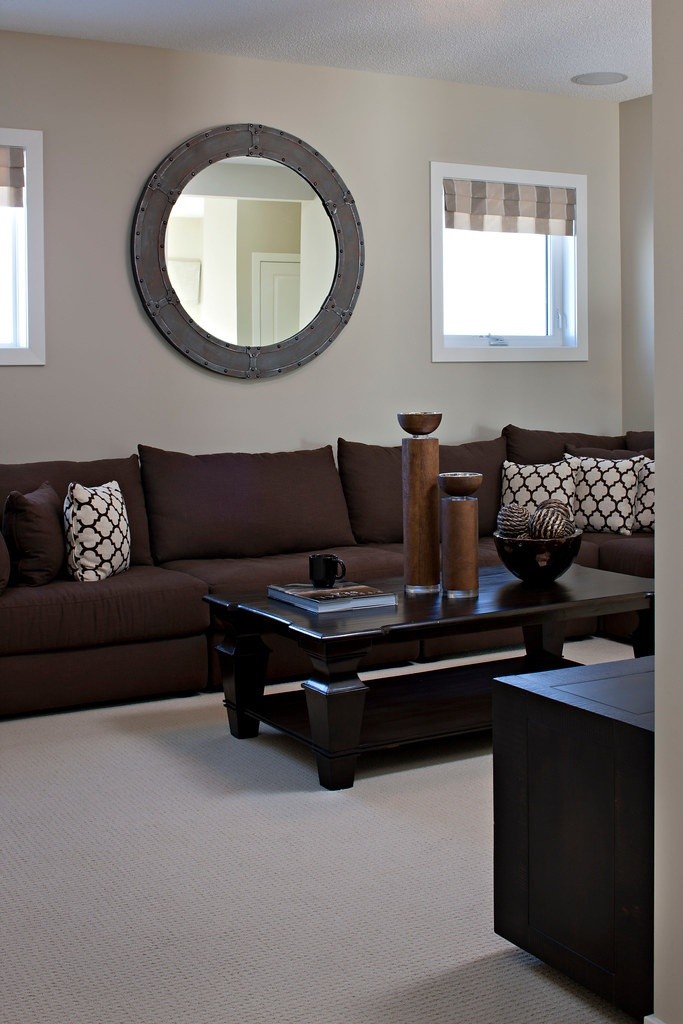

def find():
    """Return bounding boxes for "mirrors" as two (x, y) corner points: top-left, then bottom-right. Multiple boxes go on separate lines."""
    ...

(0, 129), (47, 366)
(129, 124), (364, 379)
(427, 162), (589, 363)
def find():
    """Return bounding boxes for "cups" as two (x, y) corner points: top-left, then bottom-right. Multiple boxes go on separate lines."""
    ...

(309, 553), (345, 587)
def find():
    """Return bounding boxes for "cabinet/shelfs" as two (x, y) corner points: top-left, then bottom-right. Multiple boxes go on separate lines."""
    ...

(484, 659), (655, 1017)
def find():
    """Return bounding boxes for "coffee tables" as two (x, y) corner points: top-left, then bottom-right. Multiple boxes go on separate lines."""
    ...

(198, 563), (656, 789)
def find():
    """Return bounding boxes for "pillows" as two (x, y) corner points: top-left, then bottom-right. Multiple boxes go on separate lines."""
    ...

(634, 456), (656, 535)
(3, 479), (66, 587)
(1, 454), (154, 587)
(336, 437), (508, 542)
(137, 441), (358, 562)
(0, 533), (12, 594)
(562, 453), (644, 536)
(502, 424), (627, 464)
(59, 480), (132, 583)
(564, 443), (654, 460)
(497, 461), (575, 526)
(625, 430), (653, 452)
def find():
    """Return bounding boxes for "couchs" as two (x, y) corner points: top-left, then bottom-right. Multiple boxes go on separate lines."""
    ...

(0, 426), (657, 719)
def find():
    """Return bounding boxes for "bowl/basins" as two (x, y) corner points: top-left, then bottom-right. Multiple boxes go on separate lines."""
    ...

(492, 529), (583, 583)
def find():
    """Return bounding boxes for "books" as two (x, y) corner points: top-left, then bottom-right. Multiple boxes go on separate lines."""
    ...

(265, 580), (398, 614)
